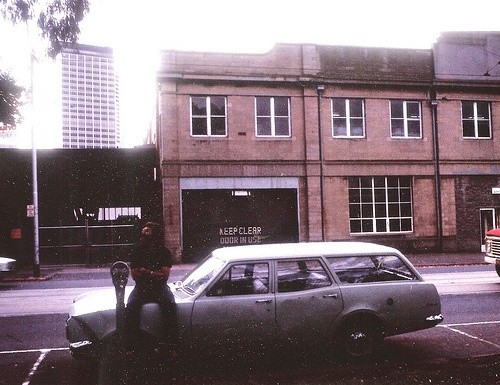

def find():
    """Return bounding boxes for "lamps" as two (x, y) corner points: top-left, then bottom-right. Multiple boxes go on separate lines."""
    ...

(431, 100), (437, 106)
(318, 84), (325, 90)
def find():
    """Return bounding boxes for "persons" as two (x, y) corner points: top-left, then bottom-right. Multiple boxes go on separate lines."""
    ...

(127, 222), (177, 357)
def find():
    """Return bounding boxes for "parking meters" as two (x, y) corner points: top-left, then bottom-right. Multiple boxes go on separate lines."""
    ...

(110, 260), (129, 364)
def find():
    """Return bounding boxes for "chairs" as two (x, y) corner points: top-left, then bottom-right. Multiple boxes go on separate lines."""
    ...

(302, 273), (331, 290)
(251, 280), (268, 295)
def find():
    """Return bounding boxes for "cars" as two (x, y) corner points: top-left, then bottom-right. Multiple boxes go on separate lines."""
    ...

(64, 241), (444, 366)
